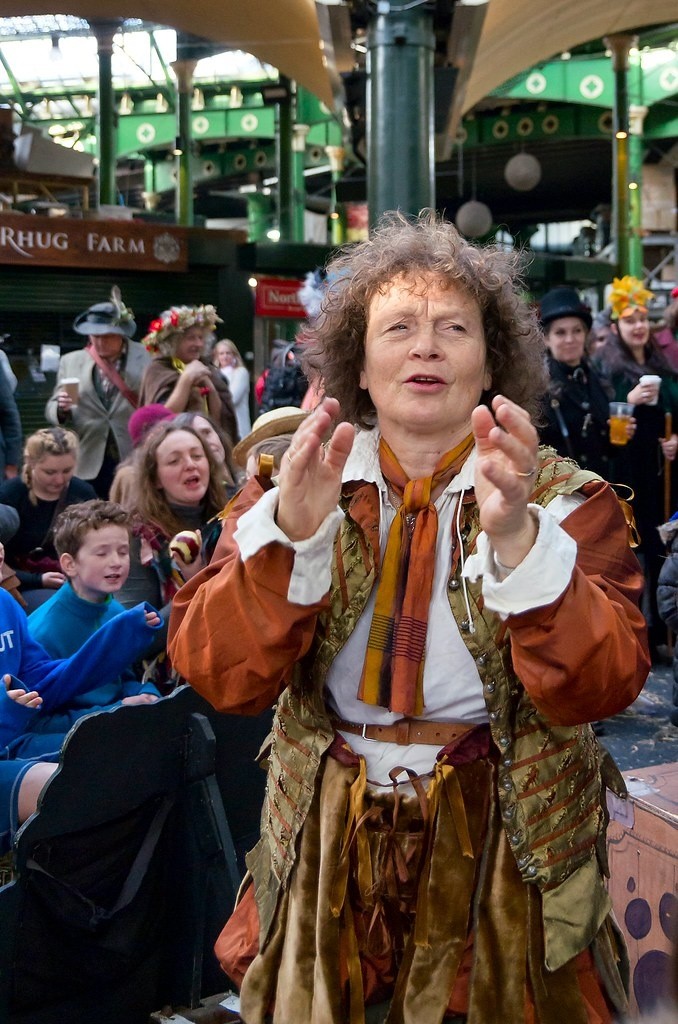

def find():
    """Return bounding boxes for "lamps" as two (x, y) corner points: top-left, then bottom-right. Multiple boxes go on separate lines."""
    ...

(118, 92), (133, 114)
(191, 88), (207, 110)
(41, 98), (53, 120)
(502, 141), (542, 190)
(453, 148), (492, 238)
(83, 95), (93, 117)
(228, 86), (245, 109)
(155, 93), (170, 114)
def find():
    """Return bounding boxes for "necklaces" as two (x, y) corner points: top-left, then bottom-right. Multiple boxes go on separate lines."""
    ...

(388, 482), (399, 509)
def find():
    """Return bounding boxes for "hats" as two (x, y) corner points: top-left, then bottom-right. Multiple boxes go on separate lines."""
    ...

(142, 304), (218, 351)
(609, 275), (654, 319)
(535, 288), (592, 333)
(231, 406), (331, 471)
(73, 285), (136, 337)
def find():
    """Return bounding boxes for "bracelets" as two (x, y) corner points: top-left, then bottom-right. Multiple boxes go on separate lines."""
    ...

(493, 551), (514, 573)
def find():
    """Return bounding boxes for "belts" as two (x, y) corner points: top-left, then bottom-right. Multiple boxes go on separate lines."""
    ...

(326, 707), (480, 746)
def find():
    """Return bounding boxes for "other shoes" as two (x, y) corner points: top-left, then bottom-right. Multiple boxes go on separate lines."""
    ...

(590, 720), (604, 733)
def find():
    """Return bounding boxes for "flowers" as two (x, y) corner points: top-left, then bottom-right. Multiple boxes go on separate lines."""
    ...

(141, 304), (225, 356)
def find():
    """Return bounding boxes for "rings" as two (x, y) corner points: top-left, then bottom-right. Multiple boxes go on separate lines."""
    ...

(286, 453), (290, 460)
(510, 460), (537, 476)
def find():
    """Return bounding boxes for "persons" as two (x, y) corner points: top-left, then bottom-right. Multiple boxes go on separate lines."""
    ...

(0, 351), (23, 481)
(109, 405), (174, 507)
(0, 426), (97, 589)
(171, 406), (310, 581)
(27, 499), (162, 712)
(166, 221), (651, 1023)
(0, 543), (163, 858)
(535, 284), (618, 734)
(112, 424), (229, 696)
(655, 302), (678, 374)
(44, 302), (154, 502)
(591, 275), (678, 667)
(175, 414), (242, 499)
(657, 510), (678, 741)
(137, 305), (238, 447)
(212, 339), (251, 441)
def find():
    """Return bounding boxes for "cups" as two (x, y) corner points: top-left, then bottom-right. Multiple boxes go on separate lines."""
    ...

(609, 402), (635, 444)
(639, 375), (661, 405)
(61, 378), (80, 408)
